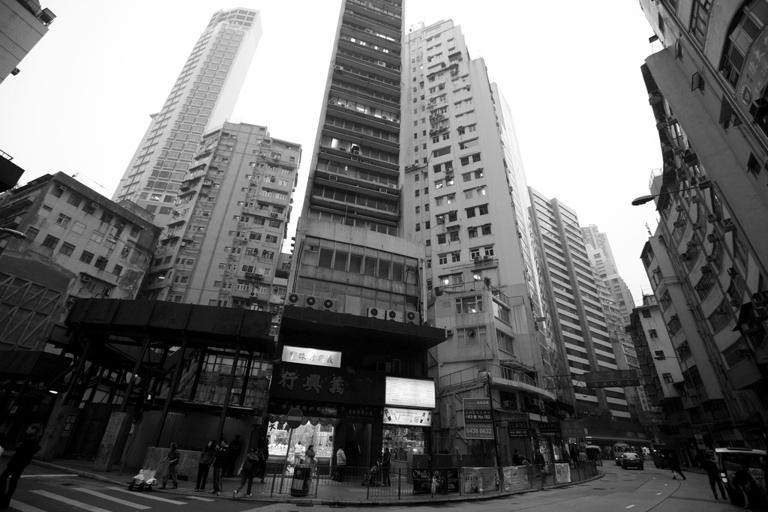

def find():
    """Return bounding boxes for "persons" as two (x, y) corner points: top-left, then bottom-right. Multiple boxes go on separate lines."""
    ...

(699, 455), (768, 512)
(381, 447), (392, 487)
(0, 423), (44, 511)
(513, 448), (530, 466)
(534, 447), (545, 470)
(668, 452), (686, 480)
(160, 442), (179, 489)
(571, 443), (580, 467)
(193, 433), (347, 500)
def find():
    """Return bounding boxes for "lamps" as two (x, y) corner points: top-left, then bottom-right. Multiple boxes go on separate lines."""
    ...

(632, 180), (712, 205)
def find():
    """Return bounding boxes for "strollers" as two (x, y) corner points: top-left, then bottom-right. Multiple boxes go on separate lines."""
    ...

(360, 459), (384, 487)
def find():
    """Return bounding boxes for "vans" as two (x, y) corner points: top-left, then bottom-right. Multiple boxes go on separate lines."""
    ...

(652, 448), (680, 469)
(715, 447), (767, 506)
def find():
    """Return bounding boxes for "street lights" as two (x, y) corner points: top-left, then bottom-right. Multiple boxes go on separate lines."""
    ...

(103, 337), (167, 470)
(480, 368), (510, 492)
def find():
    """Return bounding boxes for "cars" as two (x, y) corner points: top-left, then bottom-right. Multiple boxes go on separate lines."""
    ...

(619, 452), (644, 469)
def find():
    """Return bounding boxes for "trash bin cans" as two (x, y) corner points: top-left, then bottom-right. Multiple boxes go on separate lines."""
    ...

(291, 467), (311, 496)
(433, 454), (452, 494)
(411, 455), (431, 494)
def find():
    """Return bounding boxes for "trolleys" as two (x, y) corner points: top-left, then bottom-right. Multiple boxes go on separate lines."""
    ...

(126, 457), (175, 492)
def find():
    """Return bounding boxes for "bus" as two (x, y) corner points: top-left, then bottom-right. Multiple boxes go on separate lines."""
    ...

(611, 442), (632, 466)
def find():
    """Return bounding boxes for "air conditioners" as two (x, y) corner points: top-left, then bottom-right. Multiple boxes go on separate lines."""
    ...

(81, 275), (92, 283)
(58, 184), (67, 192)
(284, 292), (337, 313)
(246, 265), (265, 280)
(89, 201), (100, 210)
(377, 60), (386, 68)
(335, 65), (343, 71)
(379, 187), (387, 193)
(502, 401), (515, 410)
(367, 307), (421, 326)
(530, 399), (544, 410)
